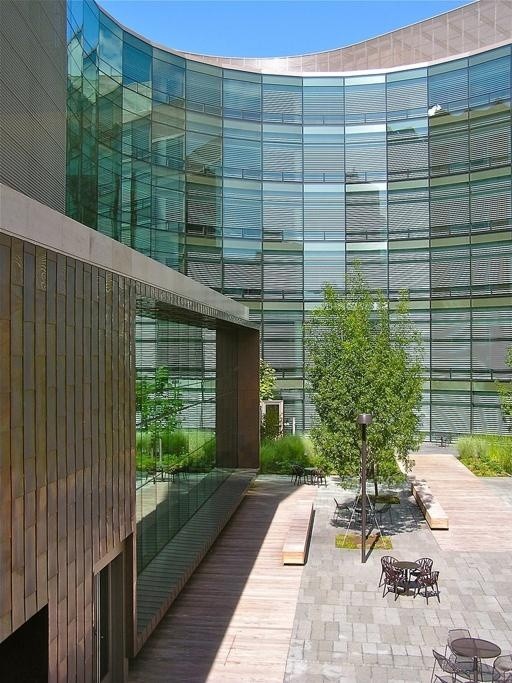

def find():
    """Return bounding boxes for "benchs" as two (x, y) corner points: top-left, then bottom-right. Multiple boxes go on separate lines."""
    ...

(282, 499), (314, 564)
(411, 480), (450, 530)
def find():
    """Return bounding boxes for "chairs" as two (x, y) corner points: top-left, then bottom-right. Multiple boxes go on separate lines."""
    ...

(291, 463), (328, 488)
(332, 495), (394, 525)
(431, 625), (511, 683)
(378, 555), (441, 605)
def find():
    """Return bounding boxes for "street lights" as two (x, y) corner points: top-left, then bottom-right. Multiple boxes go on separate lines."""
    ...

(358, 413), (372, 563)
